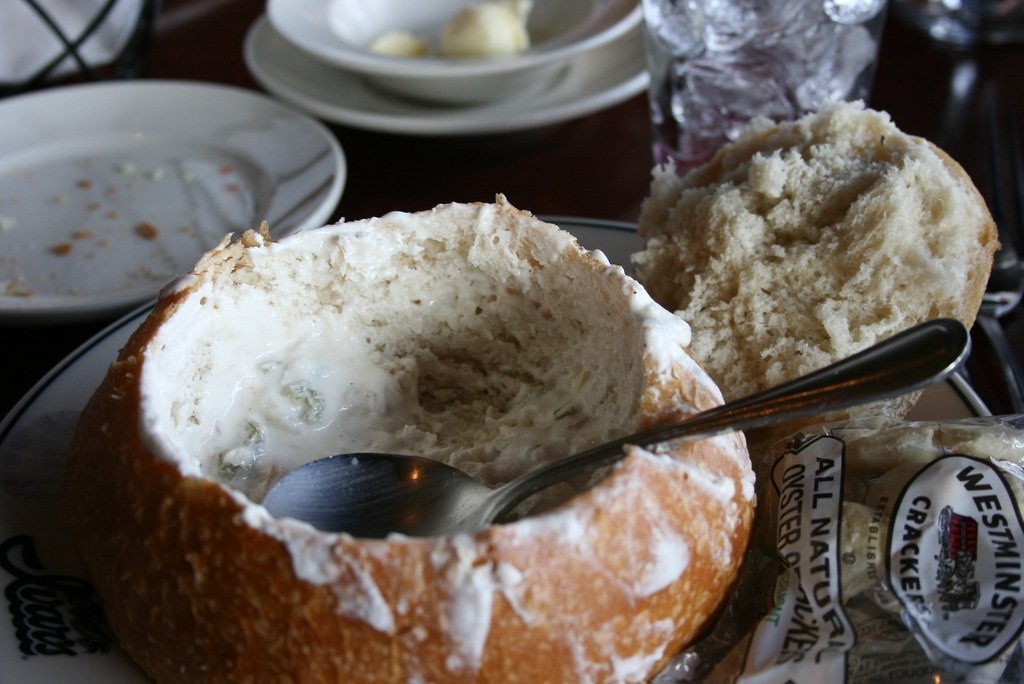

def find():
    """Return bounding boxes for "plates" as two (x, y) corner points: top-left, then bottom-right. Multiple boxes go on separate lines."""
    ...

(1, 212), (1010, 684)
(0, 79), (347, 318)
(244, 16), (651, 134)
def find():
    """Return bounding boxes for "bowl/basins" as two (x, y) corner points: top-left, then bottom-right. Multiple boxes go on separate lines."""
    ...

(265, 0), (645, 106)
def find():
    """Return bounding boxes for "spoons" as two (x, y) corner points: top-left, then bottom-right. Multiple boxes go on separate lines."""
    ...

(257, 318), (970, 540)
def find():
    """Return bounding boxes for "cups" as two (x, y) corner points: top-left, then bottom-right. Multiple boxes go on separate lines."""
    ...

(645, 0), (889, 182)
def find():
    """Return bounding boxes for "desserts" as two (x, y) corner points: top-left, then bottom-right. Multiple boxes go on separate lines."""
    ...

(437, 1), (536, 63)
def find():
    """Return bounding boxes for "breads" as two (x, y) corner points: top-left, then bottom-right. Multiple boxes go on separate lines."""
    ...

(623, 98), (1001, 438)
(64, 193), (757, 684)
